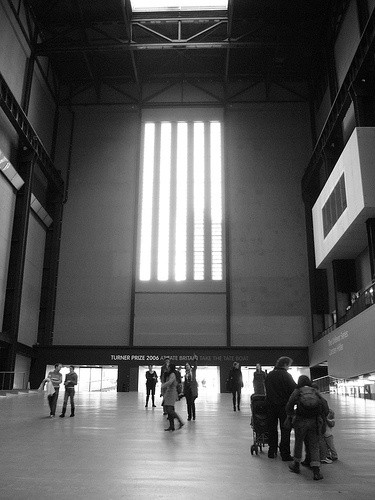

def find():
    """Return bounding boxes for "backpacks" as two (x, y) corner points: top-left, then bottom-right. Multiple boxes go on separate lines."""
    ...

(299, 387), (321, 413)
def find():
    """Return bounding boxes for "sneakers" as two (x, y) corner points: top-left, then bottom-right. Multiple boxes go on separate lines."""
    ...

(288, 461), (300, 473)
(320, 458), (333, 464)
(312, 473), (323, 480)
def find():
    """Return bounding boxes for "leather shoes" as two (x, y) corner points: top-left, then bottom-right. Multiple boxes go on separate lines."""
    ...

(176, 424), (185, 430)
(164, 427), (175, 431)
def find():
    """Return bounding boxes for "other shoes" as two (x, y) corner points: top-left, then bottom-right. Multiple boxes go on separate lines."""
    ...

(268, 449), (276, 460)
(331, 455), (340, 462)
(233, 406), (240, 411)
(280, 455), (294, 461)
(187, 415), (196, 421)
(48, 414), (75, 418)
(144, 405), (156, 407)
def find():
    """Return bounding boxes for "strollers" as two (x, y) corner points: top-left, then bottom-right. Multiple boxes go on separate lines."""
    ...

(250, 393), (277, 456)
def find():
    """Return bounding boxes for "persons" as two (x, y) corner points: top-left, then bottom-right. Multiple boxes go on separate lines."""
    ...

(253, 363), (267, 393)
(300, 382), (331, 465)
(285, 375), (323, 480)
(265, 357), (296, 461)
(46, 363), (62, 418)
(325, 410), (338, 461)
(162, 364), (184, 431)
(160, 358), (171, 414)
(228, 362), (242, 411)
(145, 364), (158, 407)
(59, 366), (78, 417)
(184, 352), (198, 421)
